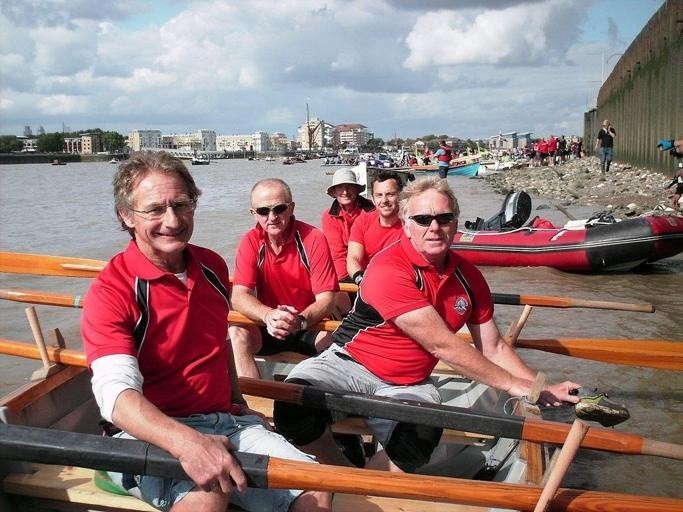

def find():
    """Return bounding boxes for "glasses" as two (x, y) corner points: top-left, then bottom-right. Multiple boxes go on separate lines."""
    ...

(253, 204), (288, 216)
(407, 212), (454, 225)
(127, 200), (197, 215)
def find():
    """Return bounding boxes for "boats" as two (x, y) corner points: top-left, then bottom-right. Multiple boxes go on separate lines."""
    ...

(0, 321), (560, 512)
(446, 189), (683, 272)
(109, 157), (120, 166)
(320, 143), (534, 177)
(51, 159), (67, 165)
(190, 142), (210, 165)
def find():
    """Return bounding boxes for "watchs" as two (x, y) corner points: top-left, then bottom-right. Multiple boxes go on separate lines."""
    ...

(298, 315), (307, 332)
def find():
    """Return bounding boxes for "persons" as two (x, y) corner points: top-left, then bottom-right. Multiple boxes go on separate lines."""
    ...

(452, 148), (476, 160)
(323, 156), (355, 165)
(595, 120), (616, 174)
(660, 138), (683, 211)
(82, 150), (333, 512)
(227, 179), (341, 379)
(273, 177), (582, 472)
(347, 170), (407, 320)
(432, 141), (453, 178)
(408, 145), (434, 167)
(322, 168), (376, 282)
(524, 135), (582, 167)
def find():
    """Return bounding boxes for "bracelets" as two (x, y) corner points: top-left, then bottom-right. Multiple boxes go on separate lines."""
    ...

(264, 307), (274, 324)
(353, 271), (363, 285)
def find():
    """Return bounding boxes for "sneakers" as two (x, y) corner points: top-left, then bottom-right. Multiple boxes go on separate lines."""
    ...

(574, 392), (631, 427)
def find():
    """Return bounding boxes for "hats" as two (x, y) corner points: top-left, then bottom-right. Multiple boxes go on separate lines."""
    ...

(325, 168), (366, 198)
(602, 120), (609, 126)
